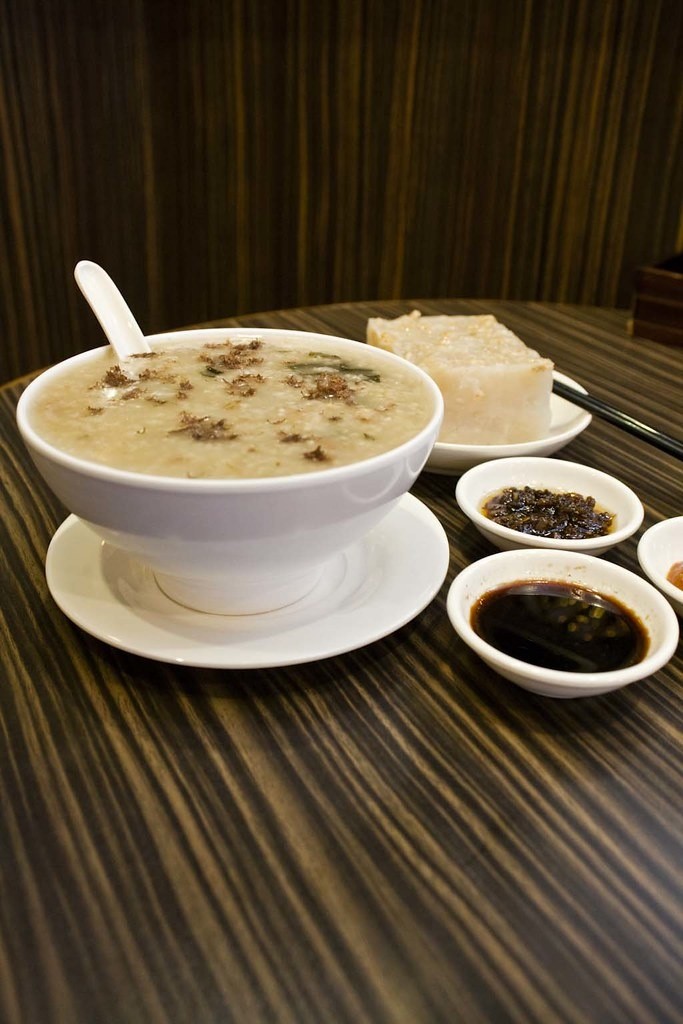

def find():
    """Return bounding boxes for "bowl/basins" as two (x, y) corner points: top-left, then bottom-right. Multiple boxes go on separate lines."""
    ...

(636, 515), (683, 619)
(455, 456), (644, 557)
(14, 326), (444, 615)
(446, 549), (679, 698)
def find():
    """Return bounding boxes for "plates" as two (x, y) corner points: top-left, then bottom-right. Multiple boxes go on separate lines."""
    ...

(43, 492), (450, 670)
(423, 369), (593, 477)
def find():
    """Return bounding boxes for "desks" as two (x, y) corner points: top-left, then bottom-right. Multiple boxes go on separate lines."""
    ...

(0, 300), (683, 1024)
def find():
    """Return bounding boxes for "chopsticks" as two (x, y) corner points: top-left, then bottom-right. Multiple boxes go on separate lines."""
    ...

(551, 379), (683, 463)
